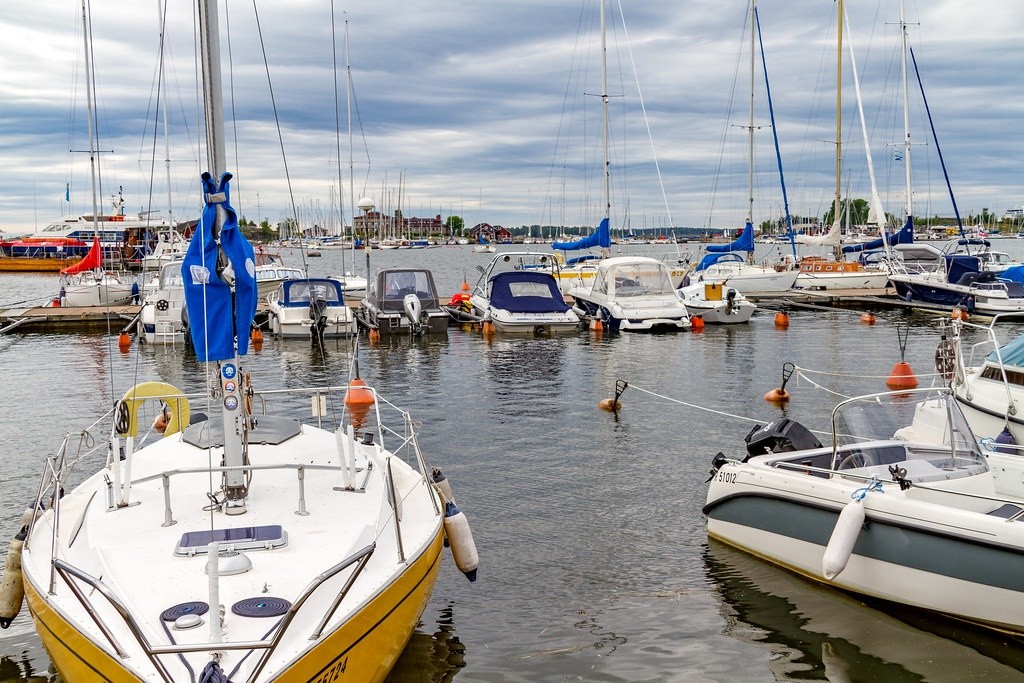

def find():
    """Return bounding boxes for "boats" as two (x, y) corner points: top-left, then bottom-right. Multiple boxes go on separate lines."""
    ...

(702, 387), (1024, 651)
(891, 308), (1024, 456)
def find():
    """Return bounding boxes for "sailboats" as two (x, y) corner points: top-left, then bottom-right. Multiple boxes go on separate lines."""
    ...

(0, 0), (479, 683)
(0, 0), (1023, 325)
